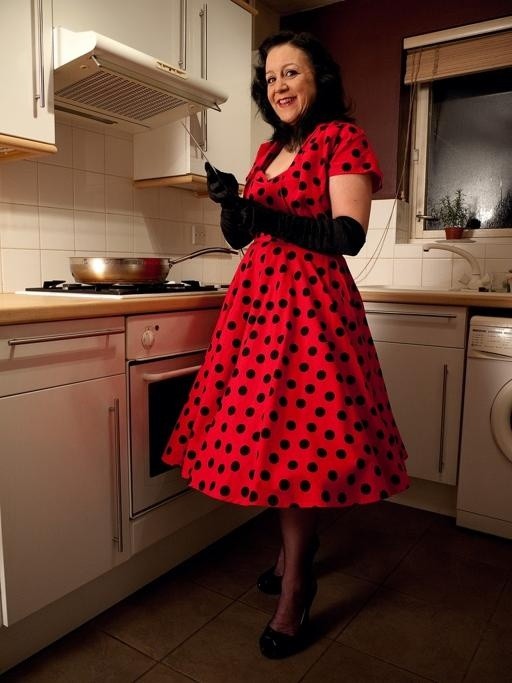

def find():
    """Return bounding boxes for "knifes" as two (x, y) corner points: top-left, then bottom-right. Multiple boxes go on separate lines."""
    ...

(180, 120), (233, 193)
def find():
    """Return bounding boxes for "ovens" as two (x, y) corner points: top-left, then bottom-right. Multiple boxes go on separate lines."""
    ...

(128, 348), (247, 556)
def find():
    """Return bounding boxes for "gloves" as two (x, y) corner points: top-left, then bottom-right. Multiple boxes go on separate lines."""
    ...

(204, 162), (366, 255)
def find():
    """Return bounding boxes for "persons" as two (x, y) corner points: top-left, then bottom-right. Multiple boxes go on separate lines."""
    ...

(160, 31), (383, 658)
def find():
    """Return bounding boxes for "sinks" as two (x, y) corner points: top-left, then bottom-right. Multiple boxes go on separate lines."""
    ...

(359, 284), (424, 292)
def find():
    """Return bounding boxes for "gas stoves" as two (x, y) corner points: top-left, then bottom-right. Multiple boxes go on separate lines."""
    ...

(15, 277), (237, 361)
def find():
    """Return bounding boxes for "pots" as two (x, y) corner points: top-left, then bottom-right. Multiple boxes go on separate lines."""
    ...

(67, 246), (239, 280)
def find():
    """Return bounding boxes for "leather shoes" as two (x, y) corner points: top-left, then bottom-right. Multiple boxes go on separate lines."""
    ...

(257, 535), (320, 594)
(259, 576), (318, 659)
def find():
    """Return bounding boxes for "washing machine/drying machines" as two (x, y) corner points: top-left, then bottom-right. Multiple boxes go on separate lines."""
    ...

(454, 315), (512, 539)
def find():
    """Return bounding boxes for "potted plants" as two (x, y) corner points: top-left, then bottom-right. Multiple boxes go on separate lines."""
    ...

(439, 188), (469, 238)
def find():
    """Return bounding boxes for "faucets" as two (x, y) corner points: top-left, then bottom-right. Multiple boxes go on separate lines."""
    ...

(423, 244), (482, 290)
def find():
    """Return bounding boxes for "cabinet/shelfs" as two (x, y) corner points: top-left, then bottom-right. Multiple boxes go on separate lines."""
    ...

(356, 290), (467, 515)
(134, 0), (258, 198)
(1, 303), (128, 628)
(0, 0), (57, 163)
